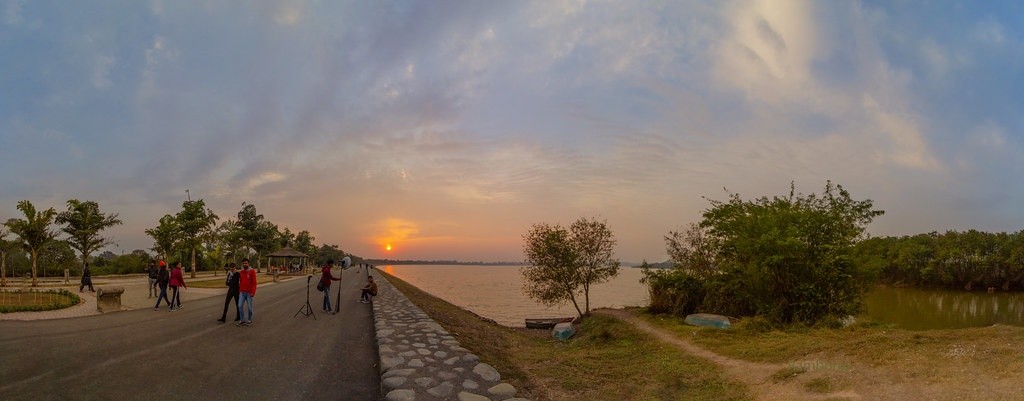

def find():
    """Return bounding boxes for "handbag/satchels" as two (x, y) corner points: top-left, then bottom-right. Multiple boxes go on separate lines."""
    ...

(317, 279), (324, 292)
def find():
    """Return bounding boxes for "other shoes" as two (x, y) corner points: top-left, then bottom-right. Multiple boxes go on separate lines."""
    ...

(234, 317), (240, 321)
(217, 318), (226, 323)
(154, 304), (184, 312)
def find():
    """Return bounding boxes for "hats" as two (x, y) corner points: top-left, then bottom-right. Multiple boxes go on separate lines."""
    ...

(159, 261), (166, 267)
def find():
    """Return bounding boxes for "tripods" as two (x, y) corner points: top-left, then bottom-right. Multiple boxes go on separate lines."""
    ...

(293, 276), (316, 320)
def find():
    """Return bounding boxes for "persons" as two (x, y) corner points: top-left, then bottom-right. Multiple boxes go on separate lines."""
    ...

(359, 263), (374, 270)
(79, 262), (96, 293)
(321, 259), (342, 315)
(272, 262), (304, 272)
(356, 276), (377, 303)
(146, 260), (188, 312)
(217, 258), (257, 326)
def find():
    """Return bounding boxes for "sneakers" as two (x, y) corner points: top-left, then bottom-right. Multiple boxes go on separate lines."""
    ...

(236, 321), (246, 326)
(246, 320), (253, 326)
